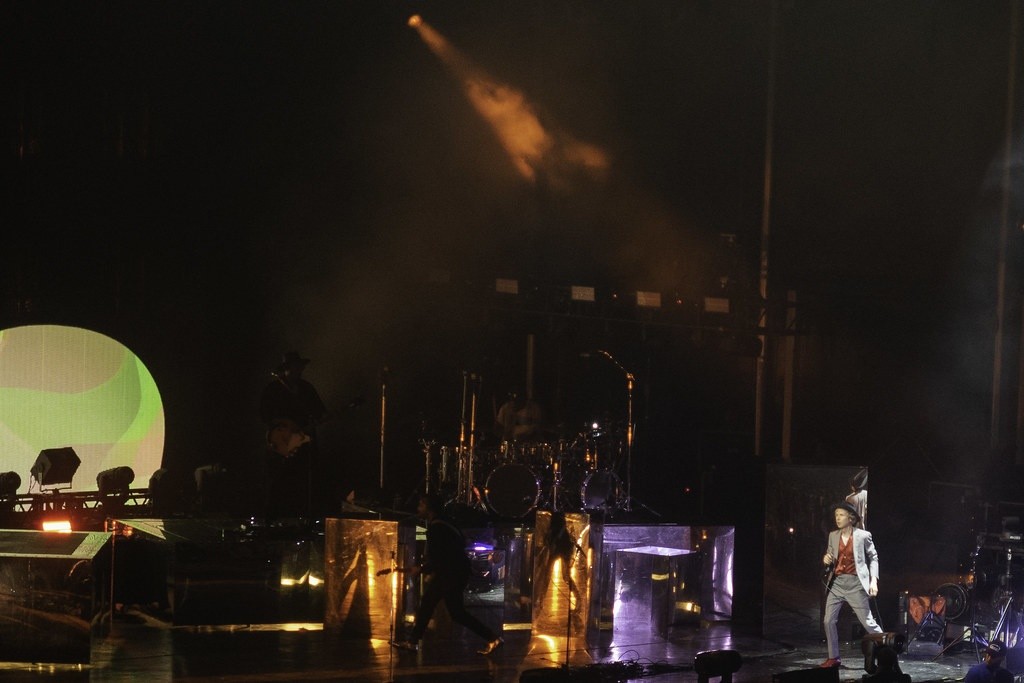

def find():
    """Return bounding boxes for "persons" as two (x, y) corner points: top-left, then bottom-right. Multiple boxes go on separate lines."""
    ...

(388, 493), (507, 656)
(821, 503), (886, 668)
(260, 351), (330, 507)
(963, 640), (1014, 682)
(861, 649), (911, 683)
(496, 385), (541, 441)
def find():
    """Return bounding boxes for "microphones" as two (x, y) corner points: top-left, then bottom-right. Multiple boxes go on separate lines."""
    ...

(825, 545), (833, 568)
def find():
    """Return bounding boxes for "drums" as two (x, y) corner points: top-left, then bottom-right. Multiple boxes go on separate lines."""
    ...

(932, 583), (968, 618)
(580, 471), (624, 516)
(484, 463), (541, 516)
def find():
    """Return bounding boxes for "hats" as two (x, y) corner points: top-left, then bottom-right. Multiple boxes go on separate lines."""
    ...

(275, 351), (311, 368)
(986, 640), (1007, 657)
(830, 500), (861, 522)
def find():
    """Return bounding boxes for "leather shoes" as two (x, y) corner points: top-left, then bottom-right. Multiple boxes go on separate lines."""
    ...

(820, 658), (841, 668)
(390, 639), (419, 654)
(477, 637), (505, 655)
